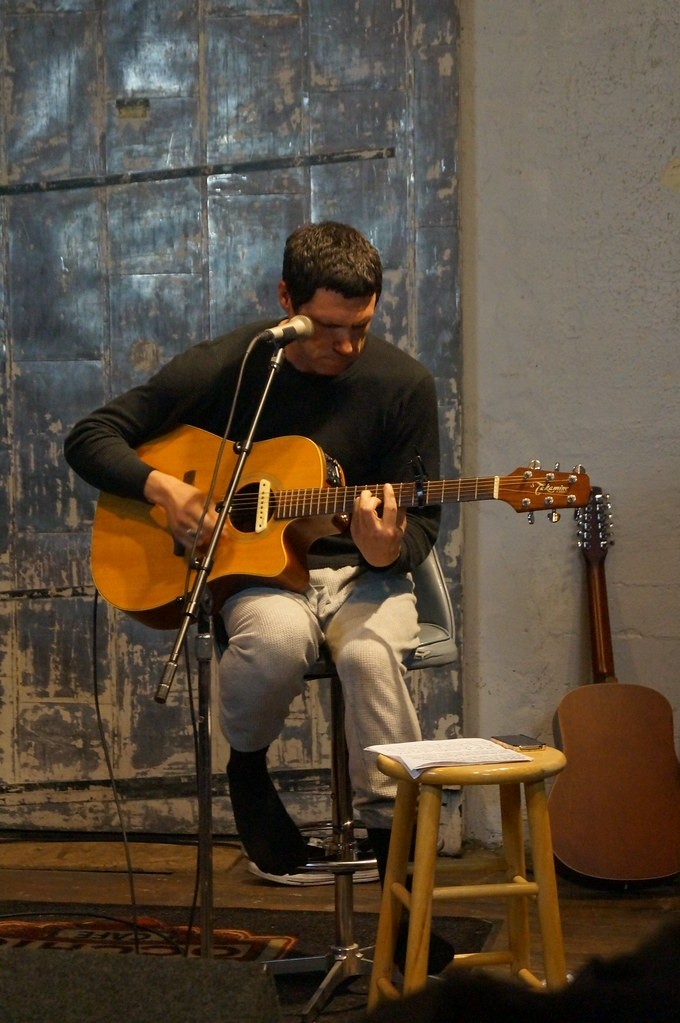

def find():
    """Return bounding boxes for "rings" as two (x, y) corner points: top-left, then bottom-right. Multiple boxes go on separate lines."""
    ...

(187, 530), (199, 539)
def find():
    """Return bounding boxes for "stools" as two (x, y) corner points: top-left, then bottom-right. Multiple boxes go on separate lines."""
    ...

(367, 743), (567, 1014)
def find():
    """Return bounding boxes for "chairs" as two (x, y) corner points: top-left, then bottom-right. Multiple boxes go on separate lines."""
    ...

(208, 542), (458, 1023)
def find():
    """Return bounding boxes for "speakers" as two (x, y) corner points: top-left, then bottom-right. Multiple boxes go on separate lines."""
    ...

(0, 945), (283, 1023)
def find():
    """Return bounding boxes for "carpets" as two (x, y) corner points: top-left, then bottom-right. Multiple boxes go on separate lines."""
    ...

(0, 899), (504, 1023)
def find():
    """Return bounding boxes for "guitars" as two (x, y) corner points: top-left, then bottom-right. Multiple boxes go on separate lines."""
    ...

(91, 424), (593, 631)
(545, 482), (678, 886)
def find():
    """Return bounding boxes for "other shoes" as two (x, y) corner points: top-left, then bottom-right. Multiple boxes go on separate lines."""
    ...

(248, 837), (335, 886)
(347, 841), (380, 881)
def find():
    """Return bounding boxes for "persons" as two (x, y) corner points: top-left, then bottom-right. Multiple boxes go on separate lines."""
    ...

(63, 221), (456, 975)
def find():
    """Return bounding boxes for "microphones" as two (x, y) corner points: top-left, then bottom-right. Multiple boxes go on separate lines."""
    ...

(259, 315), (313, 344)
